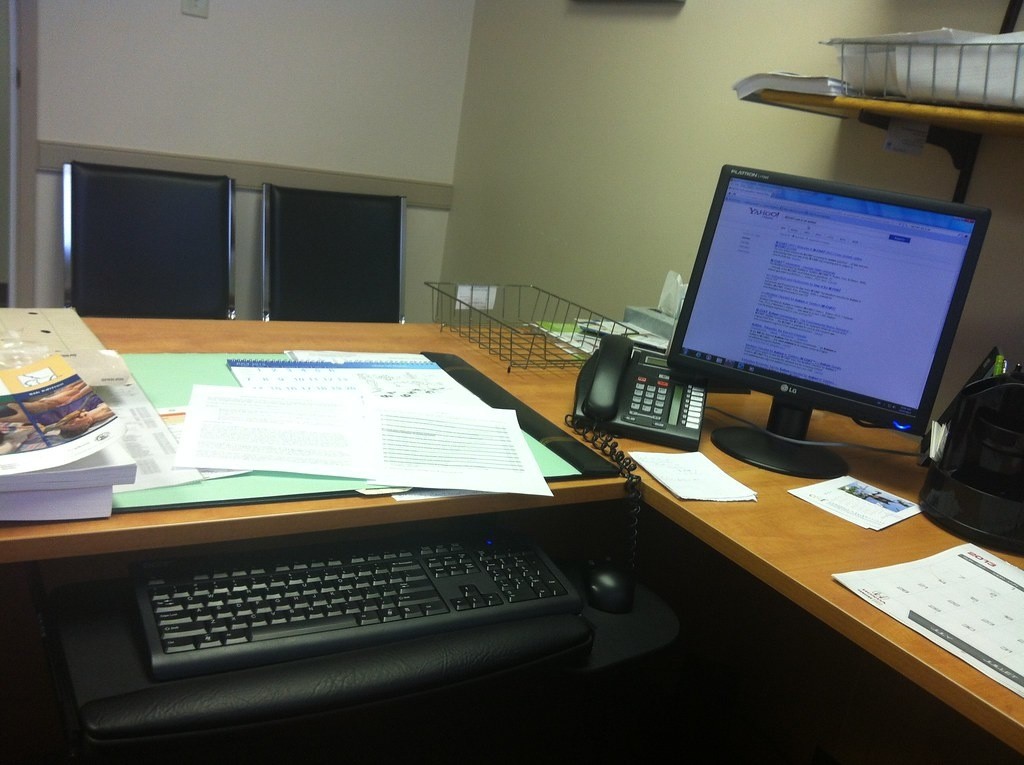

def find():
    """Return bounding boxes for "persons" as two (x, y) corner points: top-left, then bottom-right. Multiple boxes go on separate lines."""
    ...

(0, 381), (113, 456)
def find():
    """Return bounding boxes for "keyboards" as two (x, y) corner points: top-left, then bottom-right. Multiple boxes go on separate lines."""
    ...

(131, 530), (584, 684)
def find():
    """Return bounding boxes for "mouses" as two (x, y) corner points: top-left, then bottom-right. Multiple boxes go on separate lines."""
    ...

(573, 555), (648, 614)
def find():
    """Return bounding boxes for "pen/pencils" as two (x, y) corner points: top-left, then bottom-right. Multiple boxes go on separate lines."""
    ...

(993, 353), (1023, 376)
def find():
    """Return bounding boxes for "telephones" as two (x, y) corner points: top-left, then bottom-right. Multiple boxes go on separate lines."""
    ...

(571, 331), (710, 452)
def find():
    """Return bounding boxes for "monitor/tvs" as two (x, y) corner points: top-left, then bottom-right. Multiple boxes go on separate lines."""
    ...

(667, 163), (992, 478)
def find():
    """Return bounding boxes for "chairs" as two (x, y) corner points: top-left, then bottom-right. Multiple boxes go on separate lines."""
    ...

(261, 182), (406, 323)
(60, 160), (237, 321)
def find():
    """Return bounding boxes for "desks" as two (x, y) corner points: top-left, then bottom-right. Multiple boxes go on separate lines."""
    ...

(0, 312), (1020, 758)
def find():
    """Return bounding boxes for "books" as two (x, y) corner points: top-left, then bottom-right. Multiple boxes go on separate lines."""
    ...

(0, 486), (112, 519)
(0, 355), (137, 494)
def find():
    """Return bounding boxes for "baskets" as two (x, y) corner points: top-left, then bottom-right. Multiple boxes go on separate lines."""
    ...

(423, 281), (639, 373)
